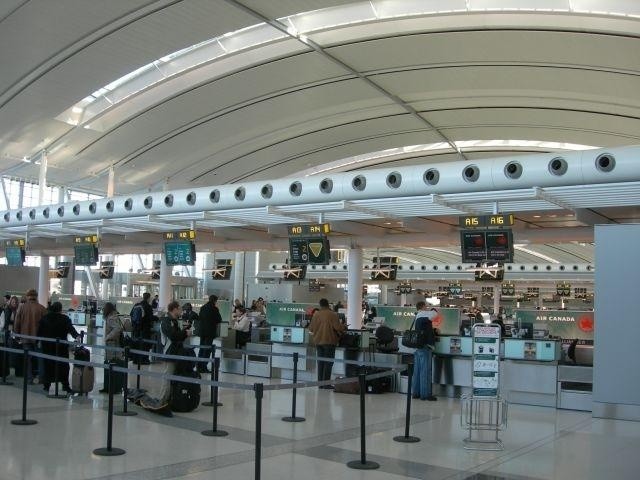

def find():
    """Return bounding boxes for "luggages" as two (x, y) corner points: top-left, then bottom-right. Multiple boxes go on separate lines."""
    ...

(72, 366), (94, 397)
(104, 359), (125, 392)
(351, 343), (384, 393)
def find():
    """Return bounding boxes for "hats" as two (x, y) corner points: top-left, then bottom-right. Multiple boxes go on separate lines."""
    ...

(182, 303), (192, 310)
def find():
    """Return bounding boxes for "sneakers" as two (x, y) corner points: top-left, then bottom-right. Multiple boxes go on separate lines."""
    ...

(43, 384), (72, 392)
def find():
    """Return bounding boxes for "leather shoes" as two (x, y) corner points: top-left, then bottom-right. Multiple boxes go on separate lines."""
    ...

(196, 368), (212, 373)
(319, 385), (334, 389)
(413, 394), (438, 402)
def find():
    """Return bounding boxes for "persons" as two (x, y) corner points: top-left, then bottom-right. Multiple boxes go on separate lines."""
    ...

(99, 293), (222, 394)
(255, 297), (266, 315)
(368, 307), (377, 318)
(411, 301), (440, 401)
(309, 298), (347, 389)
(232, 299), (250, 348)
(0, 289), (81, 394)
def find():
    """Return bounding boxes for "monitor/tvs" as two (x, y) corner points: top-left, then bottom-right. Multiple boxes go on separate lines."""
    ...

(462, 320), (484, 336)
(461, 229), (513, 281)
(284, 235), (332, 280)
(212, 259), (233, 280)
(152, 261), (160, 280)
(5, 244), (114, 280)
(372, 256), (398, 281)
(165, 240), (196, 265)
(514, 322), (534, 339)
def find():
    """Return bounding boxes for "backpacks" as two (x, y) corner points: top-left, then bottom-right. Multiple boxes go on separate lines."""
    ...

(131, 304), (145, 329)
(173, 340), (201, 412)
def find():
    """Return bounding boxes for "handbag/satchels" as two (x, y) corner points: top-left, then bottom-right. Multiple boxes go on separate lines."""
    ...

(119, 333), (135, 350)
(73, 346), (90, 366)
(402, 329), (423, 348)
(376, 326), (394, 344)
(340, 330), (360, 348)
